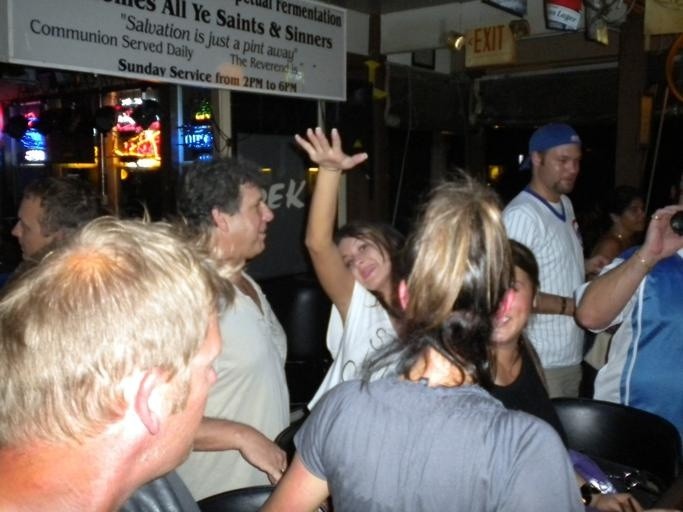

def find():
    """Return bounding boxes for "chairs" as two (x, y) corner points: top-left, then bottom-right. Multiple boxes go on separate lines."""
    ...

(541, 393), (683, 511)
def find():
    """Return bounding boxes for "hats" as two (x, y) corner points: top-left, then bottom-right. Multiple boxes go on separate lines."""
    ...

(508, 239), (538, 285)
(518, 123), (581, 171)
